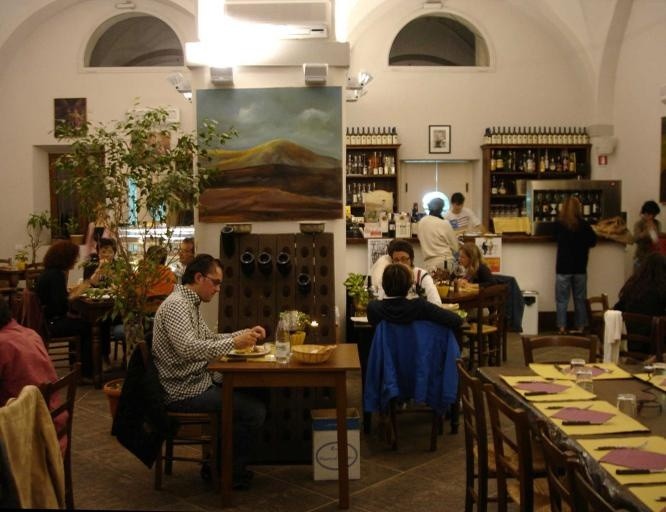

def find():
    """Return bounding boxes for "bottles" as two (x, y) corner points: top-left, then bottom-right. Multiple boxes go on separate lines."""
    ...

(490, 200), (527, 218)
(347, 152), (396, 175)
(491, 174), (498, 195)
(276, 312), (291, 362)
(388, 213), (395, 238)
(490, 148), (575, 173)
(410, 208), (418, 237)
(345, 126), (398, 145)
(498, 178), (506, 194)
(443, 260), (450, 276)
(483, 126), (589, 144)
(347, 182), (376, 203)
(535, 192), (600, 222)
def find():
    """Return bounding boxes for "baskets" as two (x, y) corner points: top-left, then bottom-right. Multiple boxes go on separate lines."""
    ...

(291, 344), (337, 364)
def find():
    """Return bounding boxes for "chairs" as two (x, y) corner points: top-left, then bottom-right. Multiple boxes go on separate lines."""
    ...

(22, 289), (82, 368)
(0, 255), (12, 265)
(585, 293), (608, 315)
(0, 385), (65, 511)
(654, 317), (666, 363)
(456, 358), (564, 512)
(569, 458), (623, 512)
(138, 341), (220, 491)
(363, 318), (459, 453)
(458, 283), (510, 368)
(25, 262), (45, 289)
(538, 418), (586, 512)
(485, 383), (552, 511)
(45, 366), (82, 512)
(521, 335), (597, 364)
(592, 311), (657, 363)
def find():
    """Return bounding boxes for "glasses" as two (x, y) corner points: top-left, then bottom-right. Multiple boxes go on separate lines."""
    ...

(202, 273), (225, 286)
(392, 256), (411, 263)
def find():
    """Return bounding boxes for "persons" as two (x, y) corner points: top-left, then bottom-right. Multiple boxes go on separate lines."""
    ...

(538, 196), (597, 335)
(367, 262), (463, 411)
(377, 241), (443, 309)
(370, 236), (409, 293)
(614, 251), (665, 362)
(413, 193), (489, 271)
(150, 251), (267, 492)
(0, 299), (69, 459)
(31, 201), (195, 386)
(449, 241), (505, 366)
(632, 199), (660, 258)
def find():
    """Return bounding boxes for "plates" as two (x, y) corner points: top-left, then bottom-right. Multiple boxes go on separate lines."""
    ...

(228, 345), (271, 356)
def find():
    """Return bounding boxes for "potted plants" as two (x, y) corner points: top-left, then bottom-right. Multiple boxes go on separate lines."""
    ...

(45, 96), (240, 422)
(344, 274), (371, 315)
(279, 310), (311, 345)
(65, 218), (85, 244)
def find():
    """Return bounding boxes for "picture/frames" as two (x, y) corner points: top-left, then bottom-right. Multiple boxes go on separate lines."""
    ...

(429, 125), (451, 154)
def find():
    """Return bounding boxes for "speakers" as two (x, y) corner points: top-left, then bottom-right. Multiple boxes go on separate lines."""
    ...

(209, 65), (236, 87)
(301, 62), (330, 85)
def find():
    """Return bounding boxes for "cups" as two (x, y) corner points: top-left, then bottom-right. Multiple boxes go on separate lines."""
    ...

(570, 358), (666, 417)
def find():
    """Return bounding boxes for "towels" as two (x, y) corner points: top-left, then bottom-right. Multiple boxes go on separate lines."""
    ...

(604, 309), (629, 369)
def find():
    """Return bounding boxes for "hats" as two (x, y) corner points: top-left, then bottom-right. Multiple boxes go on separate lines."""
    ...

(428, 198), (444, 212)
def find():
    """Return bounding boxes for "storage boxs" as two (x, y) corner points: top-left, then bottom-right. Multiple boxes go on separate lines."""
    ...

(312, 407), (360, 482)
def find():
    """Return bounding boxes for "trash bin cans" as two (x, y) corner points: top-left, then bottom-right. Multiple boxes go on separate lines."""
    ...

(518, 289), (540, 336)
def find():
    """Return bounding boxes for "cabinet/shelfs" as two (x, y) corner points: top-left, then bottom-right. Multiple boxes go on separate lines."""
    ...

(345, 143), (400, 234)
(218, 228), (335, 464)
(481, 143), (591, 232)
